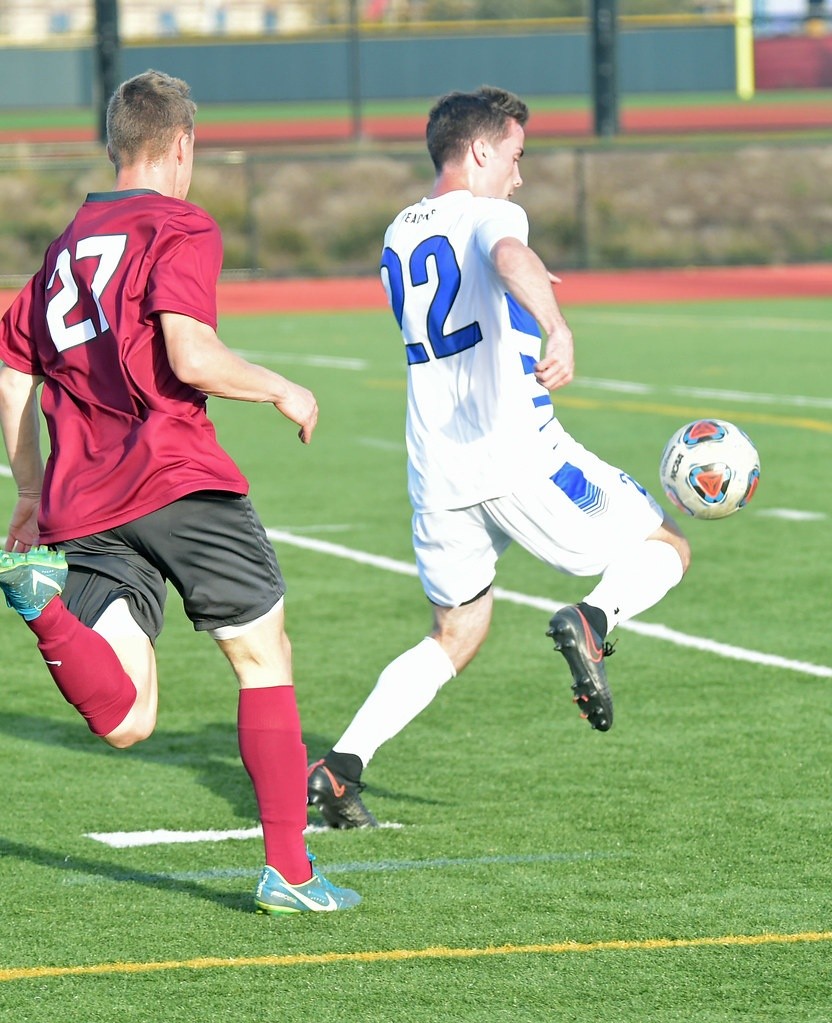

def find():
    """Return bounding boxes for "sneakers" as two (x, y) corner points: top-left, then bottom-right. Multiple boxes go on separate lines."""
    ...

(546, 602), (619, 733)
(254, 849), (362, 914)
(306, 750), (380, 831)
(0, 545), (68, 622)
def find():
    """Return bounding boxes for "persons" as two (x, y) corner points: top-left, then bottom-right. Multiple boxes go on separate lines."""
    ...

(307, 87), (691, 831)
(0, 71), (363, 915)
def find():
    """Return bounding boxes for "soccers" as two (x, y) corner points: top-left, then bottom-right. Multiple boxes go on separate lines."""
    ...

(659, 418), (761, 520)
